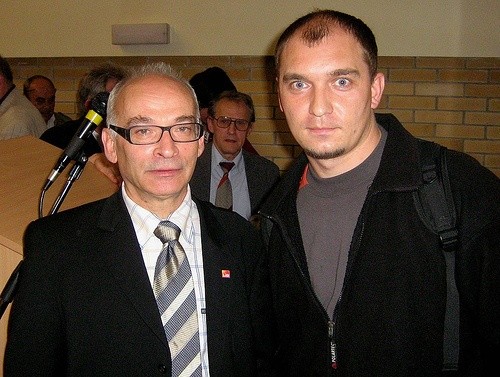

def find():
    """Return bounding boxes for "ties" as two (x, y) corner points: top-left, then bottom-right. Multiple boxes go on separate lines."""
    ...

(150, 221), (203, 377)
(215, 161), (235, 212)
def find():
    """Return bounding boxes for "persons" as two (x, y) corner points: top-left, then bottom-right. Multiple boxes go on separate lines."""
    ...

(2, 62), (275, 377)
(0, 54), (281, 220)
(248, 11), (500, 377)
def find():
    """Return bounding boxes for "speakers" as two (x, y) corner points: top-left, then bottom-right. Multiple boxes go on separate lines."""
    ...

(112, 22), (169, 46)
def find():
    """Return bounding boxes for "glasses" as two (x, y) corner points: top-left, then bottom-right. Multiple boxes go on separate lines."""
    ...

(35, 97), (56, 106)
(209, 114), (250, 132)
(109, 123), (205, 145)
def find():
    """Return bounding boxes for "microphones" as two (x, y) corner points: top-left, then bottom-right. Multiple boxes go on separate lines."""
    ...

(42, 91), (109, 191)
